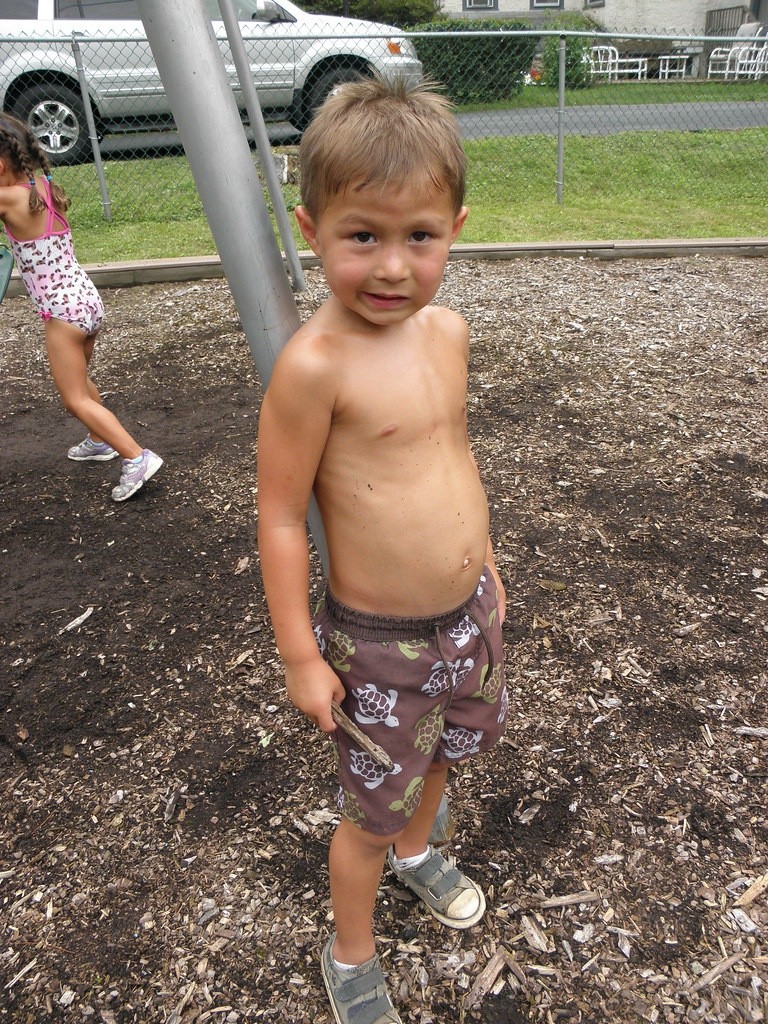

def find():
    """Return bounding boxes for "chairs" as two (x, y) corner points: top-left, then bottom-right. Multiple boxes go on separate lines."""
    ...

(581, 22), (768, 80)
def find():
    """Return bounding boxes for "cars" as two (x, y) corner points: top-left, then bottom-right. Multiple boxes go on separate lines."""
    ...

(0, 0), (426, 166)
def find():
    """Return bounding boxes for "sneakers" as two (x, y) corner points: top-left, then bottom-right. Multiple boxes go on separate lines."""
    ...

(112, 449), (164, 501)
(320, 930), (404, 1024)
(388, 842), (485, 929)
(66, 431), (118, 460)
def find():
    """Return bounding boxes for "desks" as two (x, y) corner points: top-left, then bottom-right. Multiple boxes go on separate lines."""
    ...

(656, 55), (689, 79)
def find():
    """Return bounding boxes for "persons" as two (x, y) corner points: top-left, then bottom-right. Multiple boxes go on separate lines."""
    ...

(257, 74), (507, 1022)
(1, 113), (163, 503)
